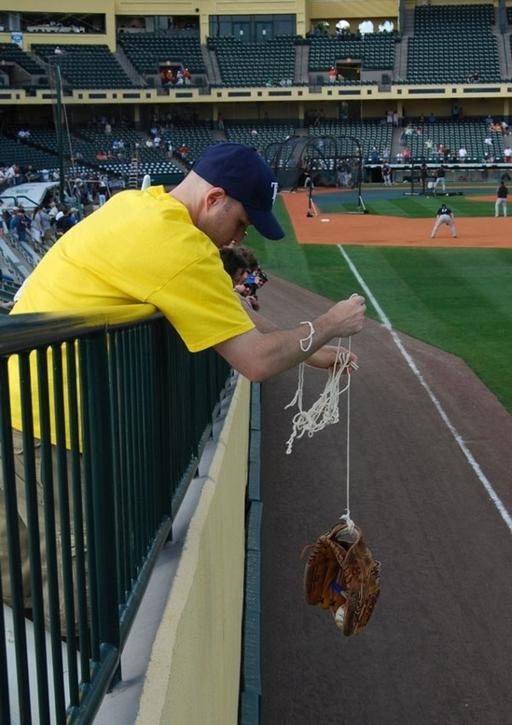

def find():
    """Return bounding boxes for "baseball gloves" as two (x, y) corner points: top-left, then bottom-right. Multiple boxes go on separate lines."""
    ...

(302, 521), (380, 636)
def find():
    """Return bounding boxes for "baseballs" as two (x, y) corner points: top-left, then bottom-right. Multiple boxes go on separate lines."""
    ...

(334, 603), (346, 632)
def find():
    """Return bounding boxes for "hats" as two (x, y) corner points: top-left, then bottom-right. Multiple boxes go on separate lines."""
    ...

(68, 208), (79, 215)
(244, 274), (258, 287)
(260, 271), (268, 281)
(192, 143), (286, 240)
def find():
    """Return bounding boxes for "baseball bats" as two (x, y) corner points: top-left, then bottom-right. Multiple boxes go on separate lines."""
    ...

(344, 212), (364, 214)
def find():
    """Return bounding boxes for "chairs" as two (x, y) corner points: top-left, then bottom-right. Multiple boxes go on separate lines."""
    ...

(1, 5), (501, 90)
(0, 114), (511, 191)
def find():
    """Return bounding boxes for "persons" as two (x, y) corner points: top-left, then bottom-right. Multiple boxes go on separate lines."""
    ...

(215, 225), (268, 312)
(493, 180), (509, 218)
(432, 167), (446, 194)
(264, 111), (271, 120)
(307, 107), (314, 119)
(249, 126), (258, 139)
(183, 68), (192, 84)
(159, 70), (168, 85)
(176, 71), (185, 85)
(166, 70), (175, 84)
(472, 72), (481, 84)
(1, 103), (190, 251)
(300, 169), (315, 190)
(466, 74), (474, 84)
(216, 118), (225, 131)
(54, 46), (64, 56)
(9, 138), (368, 665)
(328, 66), (337, 83)
(280, 76), (292, 86)
(313, 107), (320, 118)
(333, 104), (512, 191)
(313, 115), (321, 128)
(431, 203), (459, 239)
(318, 108), (326, 118)
(263, 76), (275, 87)
